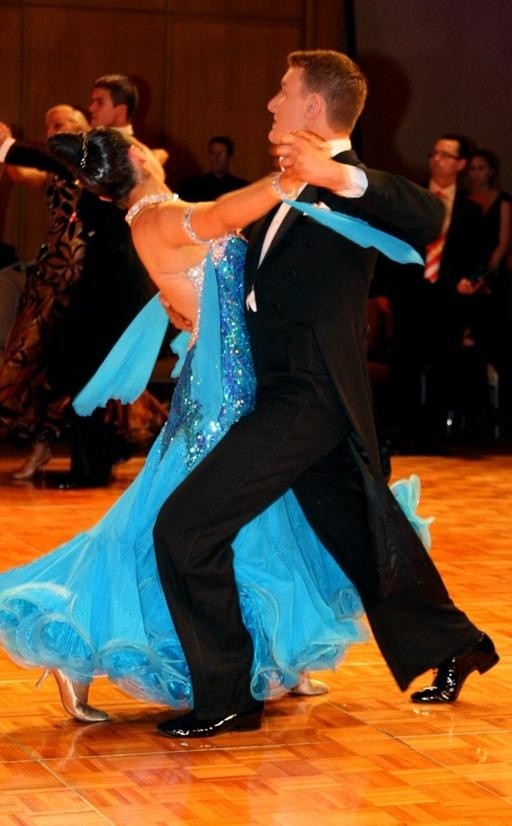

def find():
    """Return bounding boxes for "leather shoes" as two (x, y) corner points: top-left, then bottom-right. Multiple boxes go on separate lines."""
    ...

(411, 633), (499, 702)
(47, 472), (110, 489)
(157, 697), (263, 738)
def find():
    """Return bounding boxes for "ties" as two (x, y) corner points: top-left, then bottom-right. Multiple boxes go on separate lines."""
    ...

(426, 191), (444, 283)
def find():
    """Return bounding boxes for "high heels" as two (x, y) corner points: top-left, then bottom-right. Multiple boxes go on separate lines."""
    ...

(35, 665), (109, 722)
(260, 663), (328, 695)
(7, 445), (52, 480)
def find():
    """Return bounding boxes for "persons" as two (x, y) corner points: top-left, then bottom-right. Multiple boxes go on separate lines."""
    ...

(153, 47), (500, 744)
(0, 125), (336, 721)
(3, 69), (512, 487)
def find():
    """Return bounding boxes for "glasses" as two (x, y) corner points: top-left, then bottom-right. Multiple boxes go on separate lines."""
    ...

(430, 149), (459, 161)
